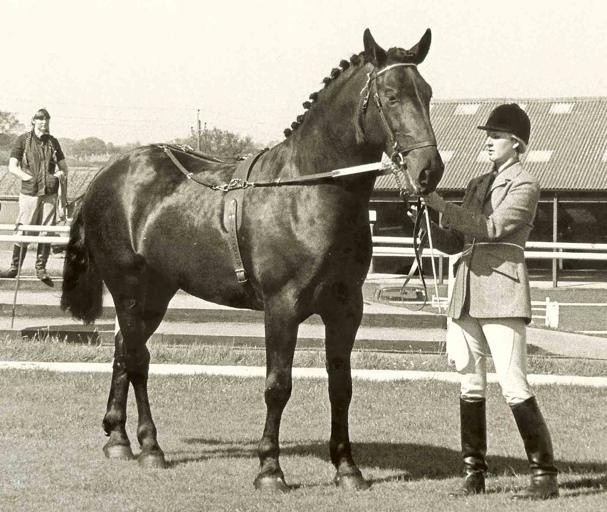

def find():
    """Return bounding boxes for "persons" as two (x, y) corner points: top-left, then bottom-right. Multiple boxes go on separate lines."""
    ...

(406, 101), (560, 501)
(1, 107), (69, 282)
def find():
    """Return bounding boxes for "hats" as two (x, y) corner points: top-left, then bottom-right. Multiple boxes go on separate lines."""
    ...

(477, 103), (530, 145)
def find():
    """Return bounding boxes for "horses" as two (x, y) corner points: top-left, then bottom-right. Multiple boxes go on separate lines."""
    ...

(59, 27), (447, 494)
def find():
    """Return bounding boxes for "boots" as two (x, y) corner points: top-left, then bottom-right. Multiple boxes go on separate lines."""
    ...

(1, 244), (27, 278)
(35, 243), (53, 280)
(450, 397), (486, 499)
(511, 396), (559, 499)
